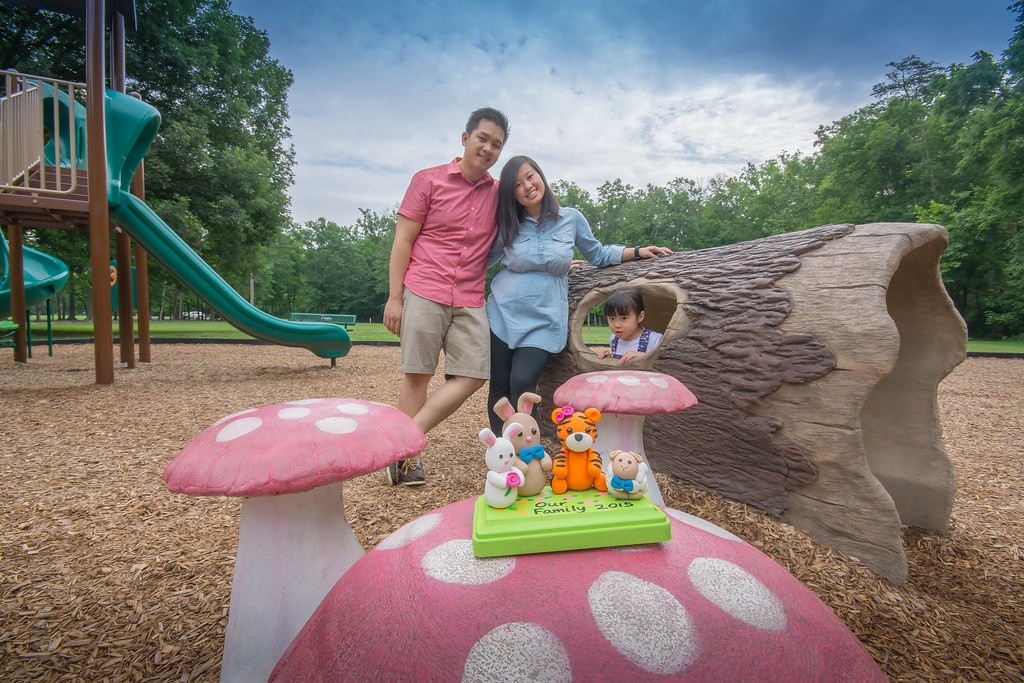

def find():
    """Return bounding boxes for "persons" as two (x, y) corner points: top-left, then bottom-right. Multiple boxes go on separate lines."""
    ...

(382, 106), (588, 488)
(480, 155), (675, 437)
(594, 287), (663, 366)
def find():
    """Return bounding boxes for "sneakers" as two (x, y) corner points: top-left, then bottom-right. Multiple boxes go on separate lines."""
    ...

(400, 455), (425, 486)
(385, 457), (406, 486)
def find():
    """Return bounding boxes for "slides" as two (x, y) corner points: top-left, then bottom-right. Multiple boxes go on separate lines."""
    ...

(0, 225), (72, 318)
(108, 190), (352, 360)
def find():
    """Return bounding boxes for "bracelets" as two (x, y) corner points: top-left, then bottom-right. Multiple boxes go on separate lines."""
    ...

(634, 245), (641, 260)
